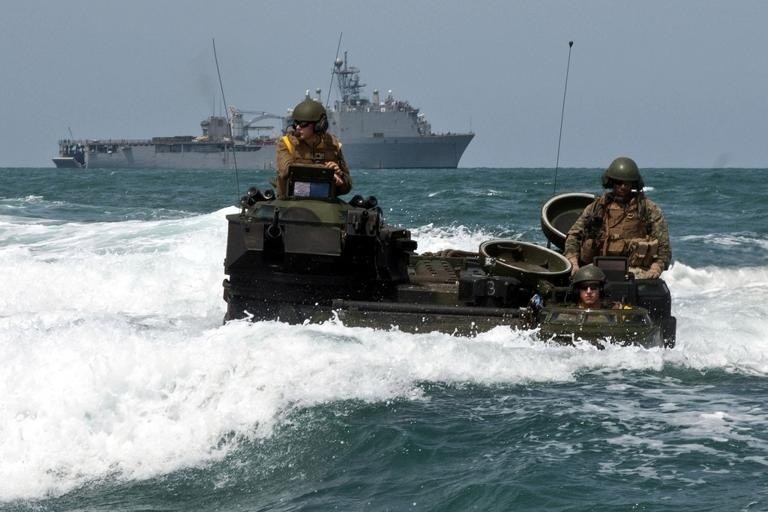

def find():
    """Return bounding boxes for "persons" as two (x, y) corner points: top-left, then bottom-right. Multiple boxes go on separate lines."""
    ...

(564, 156), (672, 280)
(276, 99), (352, 201)
(567, 265), (626, 310)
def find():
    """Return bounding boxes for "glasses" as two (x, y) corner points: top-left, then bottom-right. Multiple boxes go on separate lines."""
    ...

(580, 285), (600, 289)
(293, 121), (308, 128)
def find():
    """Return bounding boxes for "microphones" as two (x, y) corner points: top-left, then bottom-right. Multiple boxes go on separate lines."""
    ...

(291, 131), (300, 137)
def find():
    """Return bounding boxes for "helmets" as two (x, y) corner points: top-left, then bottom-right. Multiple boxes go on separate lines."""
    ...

(606, 158), (639, 181)
(571, 265), (607, 284)
(293, 101), (326, 121)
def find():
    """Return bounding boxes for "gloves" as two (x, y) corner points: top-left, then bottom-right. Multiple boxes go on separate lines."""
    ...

(566, 251), (579, 276)
(645, 262), (661, 279)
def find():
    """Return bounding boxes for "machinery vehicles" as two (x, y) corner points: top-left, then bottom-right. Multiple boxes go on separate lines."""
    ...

(212, 184), (684, 352)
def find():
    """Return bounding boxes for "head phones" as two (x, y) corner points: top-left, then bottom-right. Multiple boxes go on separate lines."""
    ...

(567, 280), (612, 298)
(314, 114), (329, 135)
(600, 175), (644, 191)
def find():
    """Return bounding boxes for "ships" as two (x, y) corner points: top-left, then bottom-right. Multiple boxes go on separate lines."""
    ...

(42, 27), (483, 173)
